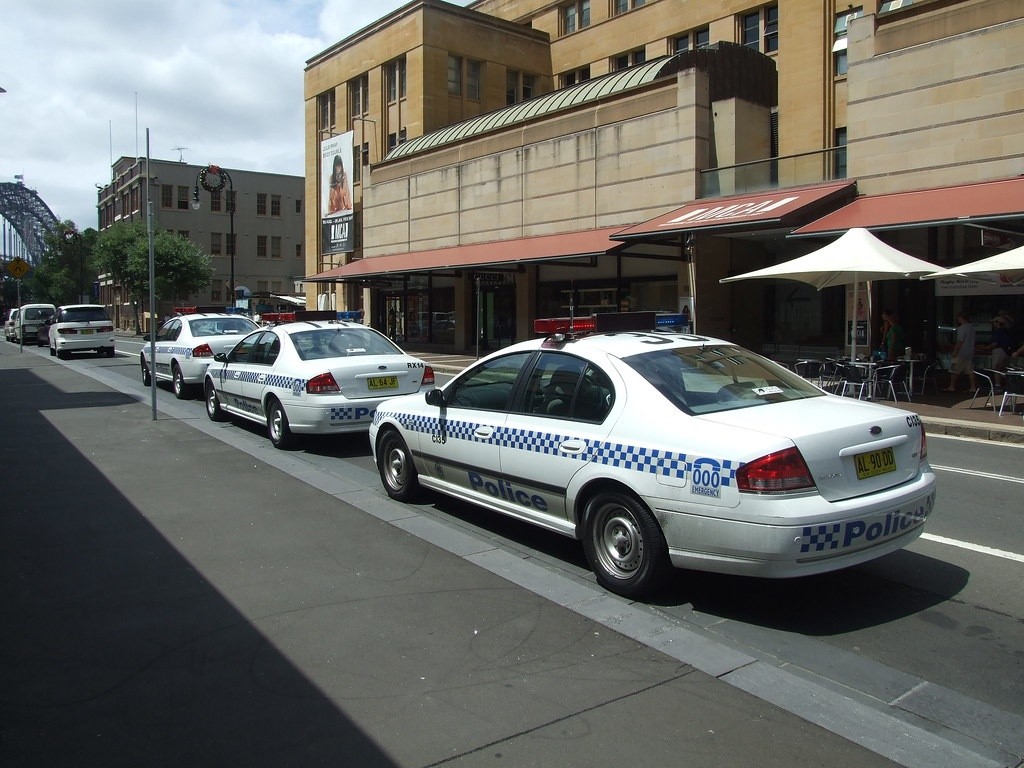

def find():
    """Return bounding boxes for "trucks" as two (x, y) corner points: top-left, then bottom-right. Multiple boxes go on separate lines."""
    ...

(263, 310), (338, 326)
(172, 306), (227, 318)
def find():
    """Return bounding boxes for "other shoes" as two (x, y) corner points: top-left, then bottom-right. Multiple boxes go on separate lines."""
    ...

(994, 385), (1002, 392)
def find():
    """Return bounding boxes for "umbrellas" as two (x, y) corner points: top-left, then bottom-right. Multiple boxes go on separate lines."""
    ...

(718, 228), (997, 362)
(920, 246), (1024, 281)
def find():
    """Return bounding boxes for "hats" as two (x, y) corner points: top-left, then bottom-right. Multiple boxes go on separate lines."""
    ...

(989, 317), (1005, 324)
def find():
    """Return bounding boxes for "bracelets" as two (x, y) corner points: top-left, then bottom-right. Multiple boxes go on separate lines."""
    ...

(882, 343), (884, 344)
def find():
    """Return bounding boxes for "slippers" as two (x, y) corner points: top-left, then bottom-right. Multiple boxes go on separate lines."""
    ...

(964, 389), (976, 394)
(940, 387), (954, 393)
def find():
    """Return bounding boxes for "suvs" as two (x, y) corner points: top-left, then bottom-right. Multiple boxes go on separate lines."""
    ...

(44, 304), (115, 359)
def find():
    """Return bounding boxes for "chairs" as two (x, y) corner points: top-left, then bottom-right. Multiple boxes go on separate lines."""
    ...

(782, 353), (1024, 416)
(545, 365), (600, 422)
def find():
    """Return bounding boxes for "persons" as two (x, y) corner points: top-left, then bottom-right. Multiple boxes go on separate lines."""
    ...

(388, 308), (396, 340)
(328, 155), (351, 215)
(879, 310), (904, 361)
(944, 313), (976, 392)
(977, 315), (1024, 395)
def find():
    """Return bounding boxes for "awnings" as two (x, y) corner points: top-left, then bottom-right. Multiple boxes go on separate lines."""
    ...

(608, 180), (855, 240)
(302, 223), (686, 283)
(785, 176), (1024, 239)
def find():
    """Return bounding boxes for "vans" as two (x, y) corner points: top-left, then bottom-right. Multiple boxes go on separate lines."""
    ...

(4, 309), (19, 343)
(9, 304), (58, 346)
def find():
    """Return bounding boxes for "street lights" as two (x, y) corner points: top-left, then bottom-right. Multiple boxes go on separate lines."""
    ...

(191, 164), (235, 310)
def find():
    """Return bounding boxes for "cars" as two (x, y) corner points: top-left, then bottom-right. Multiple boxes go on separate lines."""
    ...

(36, 315), (54, 347)
(204, 310), (434, 451)
(369, 311), (938, 608)
(433, 318), (456, 342)
(139, 306), (274, 400)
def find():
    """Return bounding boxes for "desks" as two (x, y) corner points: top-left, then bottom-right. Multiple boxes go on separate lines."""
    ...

(897, 358), (923, 396)
(1008, 370), (1024, 413)
